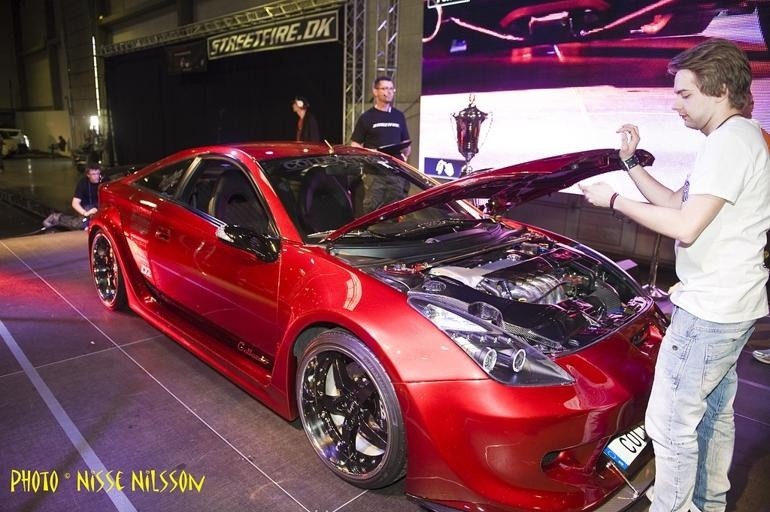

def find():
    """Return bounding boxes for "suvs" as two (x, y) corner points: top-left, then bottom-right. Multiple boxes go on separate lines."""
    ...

(0, 128), (31, 156)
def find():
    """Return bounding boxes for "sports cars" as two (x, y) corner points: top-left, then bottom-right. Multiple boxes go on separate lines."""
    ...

(84, 140), (679, 512)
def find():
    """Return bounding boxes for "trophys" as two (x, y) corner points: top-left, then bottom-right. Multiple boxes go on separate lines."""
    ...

(449, 93), (493, 178)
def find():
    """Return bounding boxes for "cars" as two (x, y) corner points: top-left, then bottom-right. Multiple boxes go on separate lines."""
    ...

(2, 136), (20, 159)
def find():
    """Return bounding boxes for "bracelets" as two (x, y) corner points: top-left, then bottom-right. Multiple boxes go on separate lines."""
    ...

(620, 156), (639, 171)
(609, 193), (619, 210)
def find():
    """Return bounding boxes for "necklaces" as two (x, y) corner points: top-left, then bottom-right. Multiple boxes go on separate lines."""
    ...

(714, 113), (744, 128)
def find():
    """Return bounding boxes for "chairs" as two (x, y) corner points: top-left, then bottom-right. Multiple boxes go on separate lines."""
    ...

(298, 166), (355, 230)
(207, 170), (270, 243)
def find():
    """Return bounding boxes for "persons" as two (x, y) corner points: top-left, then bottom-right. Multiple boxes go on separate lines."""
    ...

(59, 136), (66, 151)
(290, 94), (320, 142)
(752, 229), (770, 364)
(577, 36), (769, 511)
(349, 76), (412, 224)
(42, 164), (102, 230)
(0, 134), (7, 176)
